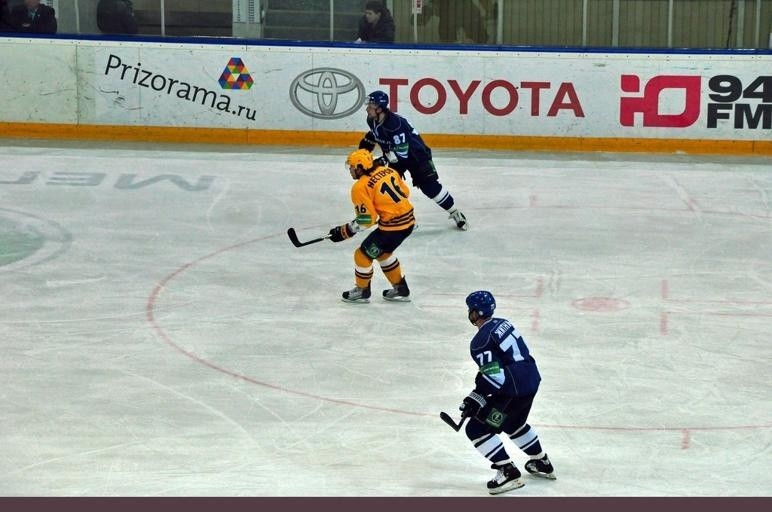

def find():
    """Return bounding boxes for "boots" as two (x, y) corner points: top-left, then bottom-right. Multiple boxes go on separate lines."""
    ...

(487, 462), (521, 488)
(525, 454), (553, 474)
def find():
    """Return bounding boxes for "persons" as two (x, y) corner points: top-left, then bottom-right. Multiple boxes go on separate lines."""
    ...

(8, 0), (57, 35)
(359, 90), (465, 229)
(97, 0), (138, 34)
(328, 148), (416, 301)
(459, 290), (554, 488)
(357, 1), (395, 44)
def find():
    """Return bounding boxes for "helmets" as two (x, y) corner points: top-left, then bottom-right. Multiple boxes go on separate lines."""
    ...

(345, 150), (374, 171)
(466, 291), (495, 324)
(365, 91), (389, 108)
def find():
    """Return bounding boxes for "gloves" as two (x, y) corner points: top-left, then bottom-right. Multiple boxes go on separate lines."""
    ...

(459, 392), (487, 416)
(328, 224), (356, 242)
(358, 132), (375, 149)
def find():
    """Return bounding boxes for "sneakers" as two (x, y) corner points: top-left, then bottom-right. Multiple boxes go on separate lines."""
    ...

(342, 280), (371, 301)
(452, 210), (467, 228)
(383, 276), (409, 298)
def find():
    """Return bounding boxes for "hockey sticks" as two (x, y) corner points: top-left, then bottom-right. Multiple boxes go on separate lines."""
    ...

(288, 228), (333, 247)
(440, 412), (467, 432)
